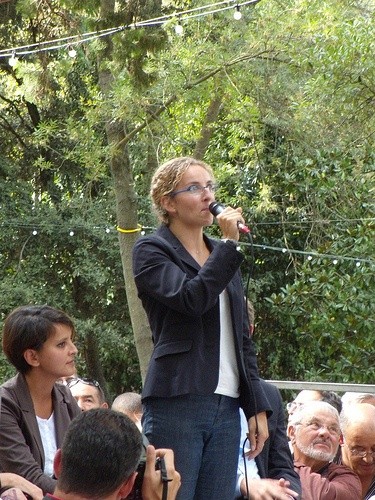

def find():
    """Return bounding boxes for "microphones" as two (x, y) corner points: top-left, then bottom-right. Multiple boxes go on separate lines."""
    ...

(209, 202), (250, 234)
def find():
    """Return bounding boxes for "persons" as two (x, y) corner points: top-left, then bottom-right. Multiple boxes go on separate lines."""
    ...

(133, 156), (273, 500)
(232, 297), (302, 500)
(0, 306), (83, 493)
(340, 402), (375, 500)
(64, 373), (143, 433)
(287, 400), (363, 500)
(285, 389), (375, 466)
(0, 407), (181, 500)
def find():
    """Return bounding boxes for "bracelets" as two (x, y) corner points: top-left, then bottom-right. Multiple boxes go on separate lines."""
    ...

(221, 238), (240, 252)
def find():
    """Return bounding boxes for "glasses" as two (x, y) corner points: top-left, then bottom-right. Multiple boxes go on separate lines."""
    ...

(67, 377), (103, 393)
(295, 422), (341, 436)
(287, 402), (304, 411)
(344, 439), (375, 458)
(170, 183), (220, 197)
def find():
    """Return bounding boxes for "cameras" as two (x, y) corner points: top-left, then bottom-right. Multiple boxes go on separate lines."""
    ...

(132, 457), (161, 494)
